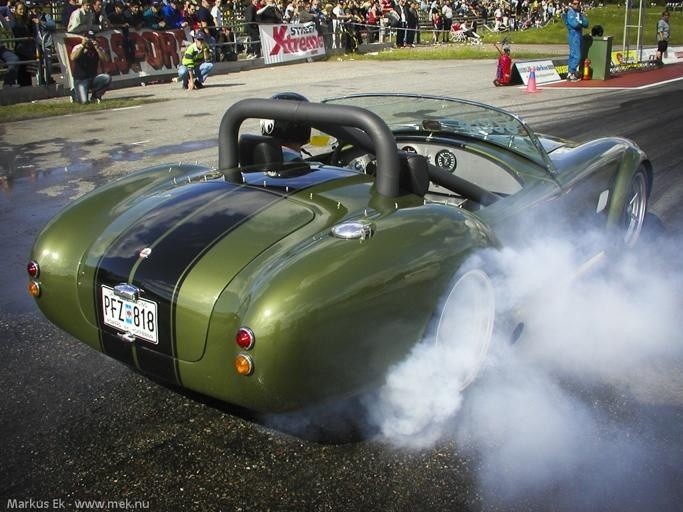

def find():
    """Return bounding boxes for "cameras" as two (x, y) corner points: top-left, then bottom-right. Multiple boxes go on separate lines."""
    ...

(88, 38), (94, 47)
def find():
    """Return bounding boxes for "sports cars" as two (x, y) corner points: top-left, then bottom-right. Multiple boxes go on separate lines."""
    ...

(28, 91), (653, 416)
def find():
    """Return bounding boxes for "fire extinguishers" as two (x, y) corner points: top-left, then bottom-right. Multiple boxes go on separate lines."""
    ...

(494, 48), (513, 86)
(583, 58), (591, 80)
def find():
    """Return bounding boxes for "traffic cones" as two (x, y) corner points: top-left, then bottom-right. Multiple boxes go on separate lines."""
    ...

(522, 69), (541, 93)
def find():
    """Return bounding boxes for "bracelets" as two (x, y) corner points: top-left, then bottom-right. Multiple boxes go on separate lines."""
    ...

(80, 45), (85, 49)
(95, 48), (100, 53)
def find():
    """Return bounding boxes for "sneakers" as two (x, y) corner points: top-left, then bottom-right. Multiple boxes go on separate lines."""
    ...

(567, 73), (580, 82)
(89, 95), (101, 104)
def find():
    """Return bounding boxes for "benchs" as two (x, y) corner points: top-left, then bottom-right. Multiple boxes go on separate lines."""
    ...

(412, 7), (510, 47)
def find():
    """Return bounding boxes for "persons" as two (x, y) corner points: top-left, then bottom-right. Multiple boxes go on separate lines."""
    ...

(0, 0), (598, 90)
(258, 90), (314, 163)
(68, 29), (113, 105)
(655, 11), (672, 66)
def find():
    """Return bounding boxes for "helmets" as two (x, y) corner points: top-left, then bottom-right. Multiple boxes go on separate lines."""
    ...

(592, 25), (603, 36)
(260, 92), (310, 146)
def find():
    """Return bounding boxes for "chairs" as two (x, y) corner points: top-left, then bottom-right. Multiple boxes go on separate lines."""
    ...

(399, 149), (432, 198)
(236, 133), (285, 172)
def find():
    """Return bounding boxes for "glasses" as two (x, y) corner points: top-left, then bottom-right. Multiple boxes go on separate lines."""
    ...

(572, 3), (580, 5)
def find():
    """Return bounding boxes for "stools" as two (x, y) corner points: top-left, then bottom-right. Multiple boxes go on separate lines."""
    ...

(610, 55), (657, 78)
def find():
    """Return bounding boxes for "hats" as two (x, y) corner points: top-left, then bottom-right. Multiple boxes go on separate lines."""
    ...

(83, 29), (95, 38)
(193, 31), (205, 39)
